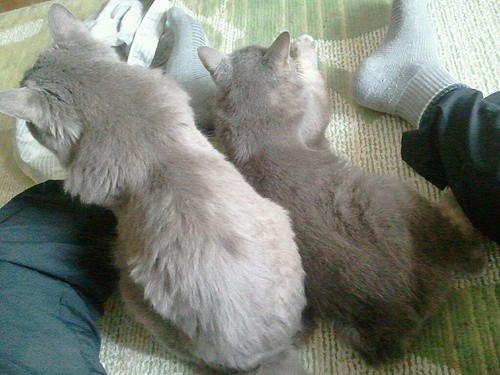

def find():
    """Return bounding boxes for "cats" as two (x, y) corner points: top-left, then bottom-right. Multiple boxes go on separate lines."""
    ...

(0, 2), (312, 375)
(197, 31), (487, 367)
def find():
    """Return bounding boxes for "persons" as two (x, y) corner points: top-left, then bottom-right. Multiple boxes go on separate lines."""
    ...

(0, 1), (500, 375)
